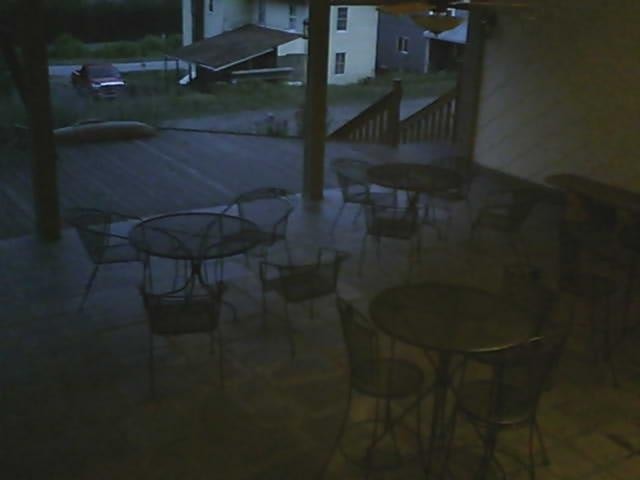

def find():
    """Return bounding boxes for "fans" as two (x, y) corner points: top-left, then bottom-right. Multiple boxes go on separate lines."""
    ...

(375, 0), (526, 16)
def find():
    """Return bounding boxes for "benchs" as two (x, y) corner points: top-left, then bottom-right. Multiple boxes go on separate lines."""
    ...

(546, 173), (639, 247)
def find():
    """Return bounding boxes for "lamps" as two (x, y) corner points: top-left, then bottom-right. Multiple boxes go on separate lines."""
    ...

(409, 10), (465, 37)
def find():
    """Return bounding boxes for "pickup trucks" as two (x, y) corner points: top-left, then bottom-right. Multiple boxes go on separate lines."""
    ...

(71, 63), (128, 98)
